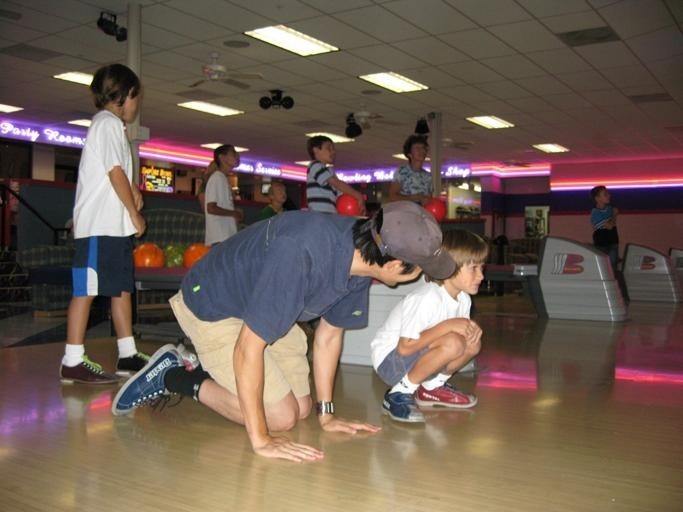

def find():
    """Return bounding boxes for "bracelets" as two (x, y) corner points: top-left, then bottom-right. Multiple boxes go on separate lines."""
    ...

(314, 400), (335, 415)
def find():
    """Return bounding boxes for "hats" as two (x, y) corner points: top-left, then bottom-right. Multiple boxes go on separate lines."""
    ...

(371, 199), (457, 279)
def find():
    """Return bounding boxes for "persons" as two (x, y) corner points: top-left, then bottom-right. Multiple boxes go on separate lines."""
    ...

(390, 133), (434, 206)
(104, 198), (458, 465)
(586, 181), (620, 278)
(367, 225), (492, 426)
(56, 60), (153, 387)
(254, 183), (289, 223)
(303, 135), (368, 217)
(200, 143), (243, 249)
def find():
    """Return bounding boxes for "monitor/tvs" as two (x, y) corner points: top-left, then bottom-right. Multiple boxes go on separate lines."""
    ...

(140, 165), (176, 194)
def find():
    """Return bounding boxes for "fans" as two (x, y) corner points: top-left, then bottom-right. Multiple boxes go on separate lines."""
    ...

(184, 49), (264, 92)
(439, 137), (472, 154)
(355, 113), (395, 136)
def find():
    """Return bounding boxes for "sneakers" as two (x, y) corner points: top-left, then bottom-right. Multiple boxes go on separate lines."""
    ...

(381, 388), (426, 423)
(60, 356), (119, 384)
(415, 383), (478, 409)
(112, 344), (200, 417)
(114, 353), (150, 376)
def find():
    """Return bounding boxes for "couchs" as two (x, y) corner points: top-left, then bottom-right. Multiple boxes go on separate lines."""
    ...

(18, 204), (248, 326)
(480, 236), (542, 297)
